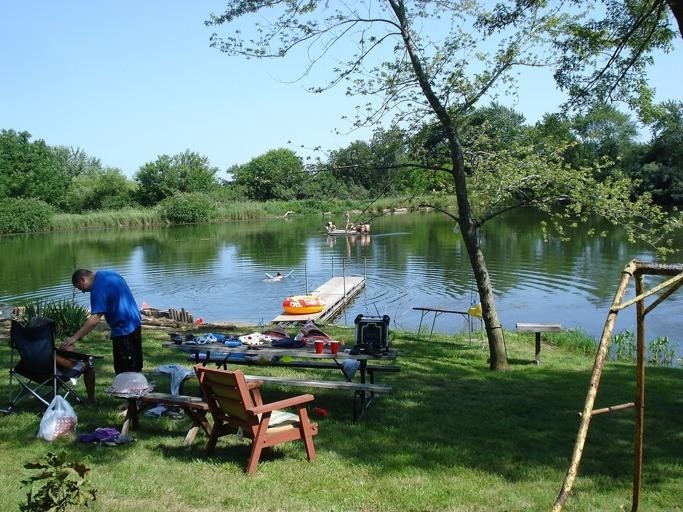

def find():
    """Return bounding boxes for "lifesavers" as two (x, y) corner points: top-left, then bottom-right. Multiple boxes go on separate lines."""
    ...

(283, 296), (325, 314)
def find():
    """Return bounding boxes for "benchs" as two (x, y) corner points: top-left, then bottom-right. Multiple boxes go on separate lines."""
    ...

(114, 393), (210, 449)
(189, 340), (401, 428)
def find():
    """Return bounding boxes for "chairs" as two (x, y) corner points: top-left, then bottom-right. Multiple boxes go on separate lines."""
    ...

(191, 362), (319, 476)
(3, 318), (98, 417)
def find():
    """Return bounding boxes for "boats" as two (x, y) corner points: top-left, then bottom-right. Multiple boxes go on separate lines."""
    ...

(327, 230), (367, 235)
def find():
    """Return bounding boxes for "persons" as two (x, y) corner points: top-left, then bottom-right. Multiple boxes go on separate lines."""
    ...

(58, 267), (143, 376)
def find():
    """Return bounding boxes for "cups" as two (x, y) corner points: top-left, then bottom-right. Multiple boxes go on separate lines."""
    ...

(315, 340), (324, 353)
(330, 341), (339, 353)
